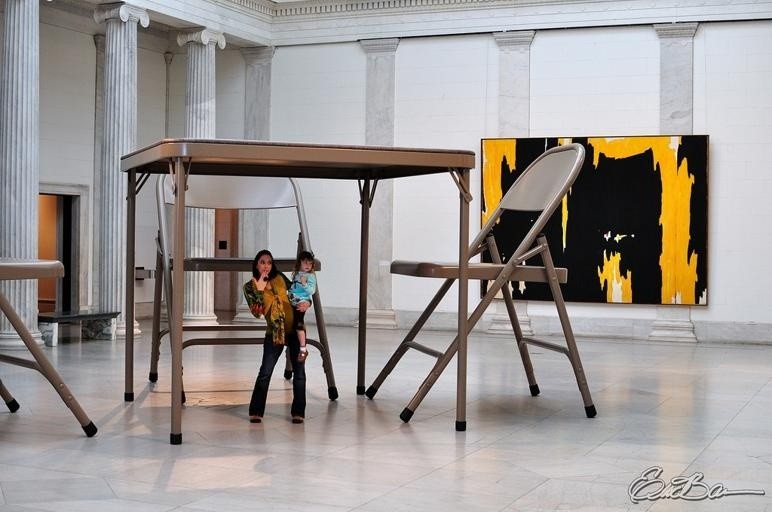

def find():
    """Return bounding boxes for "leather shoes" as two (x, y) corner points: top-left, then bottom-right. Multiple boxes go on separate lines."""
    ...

(250, 418), (261, 423)
(292, 418), (304, 424)
(297, 351), (308, 363)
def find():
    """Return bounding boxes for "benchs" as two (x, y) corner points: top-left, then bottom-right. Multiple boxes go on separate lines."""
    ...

(38, 308), (120, 346)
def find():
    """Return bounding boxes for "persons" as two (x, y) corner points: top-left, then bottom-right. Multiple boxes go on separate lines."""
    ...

(286, 251), (318, 362)
(242, 250), (314, 424)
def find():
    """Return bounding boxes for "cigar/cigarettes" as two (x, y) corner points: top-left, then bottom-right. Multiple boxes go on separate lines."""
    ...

(265, 271), (269, 278)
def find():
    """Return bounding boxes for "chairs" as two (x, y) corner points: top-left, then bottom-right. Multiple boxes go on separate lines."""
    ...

(1, 258), (97, 437)
(363, 143), (597, 423)
(149, 173), (338, 444)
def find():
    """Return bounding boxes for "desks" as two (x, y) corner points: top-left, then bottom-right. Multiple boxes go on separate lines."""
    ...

(118, 138), (475, 449)
